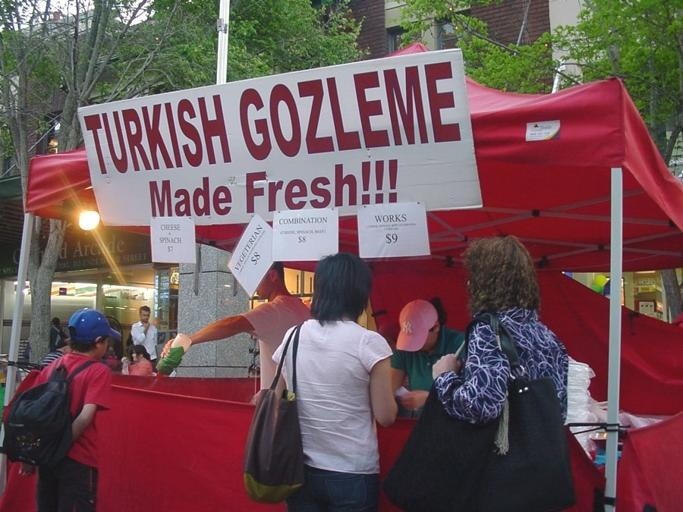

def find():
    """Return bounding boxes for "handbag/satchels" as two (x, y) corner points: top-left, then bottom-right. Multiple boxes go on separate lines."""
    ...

(240, 388), (307, 503)
(381, 375), (575, 512)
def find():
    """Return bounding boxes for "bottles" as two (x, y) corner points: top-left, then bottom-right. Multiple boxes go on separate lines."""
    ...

(154, 332), (193, 387)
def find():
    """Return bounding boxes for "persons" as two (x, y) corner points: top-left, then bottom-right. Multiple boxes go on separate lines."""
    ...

(271, 251), (398, 512)
(130, 305), (158, 364)
(50, 317), (68, 352)
(430, 234), (575, 512)
(40, 345), (71, 366)
(19, 308), (121, 512)
(160, 261), (314, 391)
(121, 344), (153, 377)
(389, 297), (468, 418)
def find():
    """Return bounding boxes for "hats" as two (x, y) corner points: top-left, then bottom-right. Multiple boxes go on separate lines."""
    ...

(68, 306), (122, 346)
(396, 299), (438, 353)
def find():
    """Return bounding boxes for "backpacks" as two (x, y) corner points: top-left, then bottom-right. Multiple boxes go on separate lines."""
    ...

(2, 364), (74, 466)
